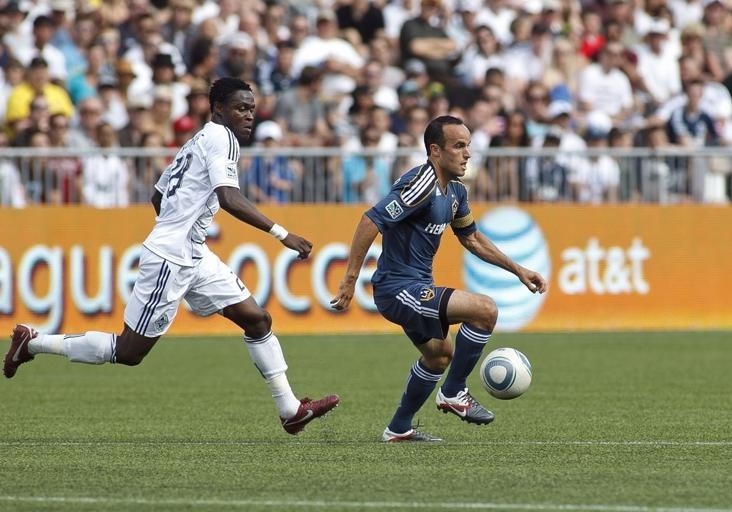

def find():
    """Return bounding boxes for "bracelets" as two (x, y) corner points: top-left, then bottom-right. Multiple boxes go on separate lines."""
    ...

(269, 219), (288, 242)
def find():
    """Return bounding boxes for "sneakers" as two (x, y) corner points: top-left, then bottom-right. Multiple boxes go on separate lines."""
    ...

(382, 430), (442, 443)
(279, 395), (339, 436)
(436, 387), (495, 426)
(2, 325), (39, 380)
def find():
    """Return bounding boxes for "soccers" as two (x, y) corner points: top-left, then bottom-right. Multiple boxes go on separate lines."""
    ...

(479, 347), (532, 400)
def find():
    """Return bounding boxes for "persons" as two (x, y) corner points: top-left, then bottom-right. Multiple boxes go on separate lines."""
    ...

(2, 77), (341, 434)
(330, 117), (547, 442)
(1, 1), (730, 203)
